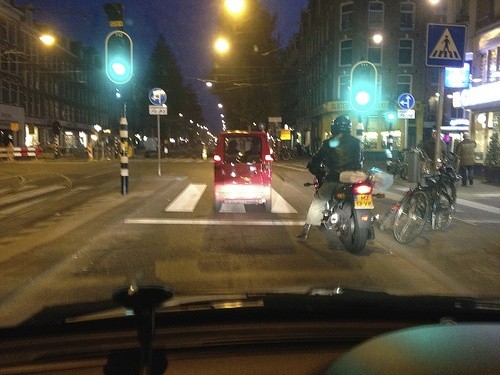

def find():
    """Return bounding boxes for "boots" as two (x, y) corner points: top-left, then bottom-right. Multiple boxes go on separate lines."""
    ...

(297, 224), (312, 239)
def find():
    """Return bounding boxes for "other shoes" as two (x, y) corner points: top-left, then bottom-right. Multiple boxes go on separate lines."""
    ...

(463, 181), (466, 185)
(470, 179), (473, 185)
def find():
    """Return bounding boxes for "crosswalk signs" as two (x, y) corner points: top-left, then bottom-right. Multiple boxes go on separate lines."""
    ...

(425, 22), (466, 68)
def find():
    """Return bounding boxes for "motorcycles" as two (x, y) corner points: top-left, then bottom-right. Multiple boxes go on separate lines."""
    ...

(214, 130), (271, 215)
(304, 160), (385, 254)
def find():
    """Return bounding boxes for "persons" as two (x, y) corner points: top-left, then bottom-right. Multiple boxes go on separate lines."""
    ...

(295, 116), (362, 240)
(418, 127), (447, 161)
(455, 132), (477, 186)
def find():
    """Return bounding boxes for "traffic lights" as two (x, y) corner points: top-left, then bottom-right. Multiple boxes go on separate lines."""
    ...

(348, 60), (378, 113)
(105, 31), (133, 84)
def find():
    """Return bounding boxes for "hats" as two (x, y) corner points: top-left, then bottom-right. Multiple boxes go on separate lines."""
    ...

(463, 132), (469, 138)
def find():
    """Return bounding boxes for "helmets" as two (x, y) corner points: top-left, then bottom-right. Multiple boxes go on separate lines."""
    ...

(331, 115), (351, 135)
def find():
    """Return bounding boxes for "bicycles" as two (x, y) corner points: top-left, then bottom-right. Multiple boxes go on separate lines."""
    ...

(378, 156), (463, 244)
(273, 148), (299, 162)
